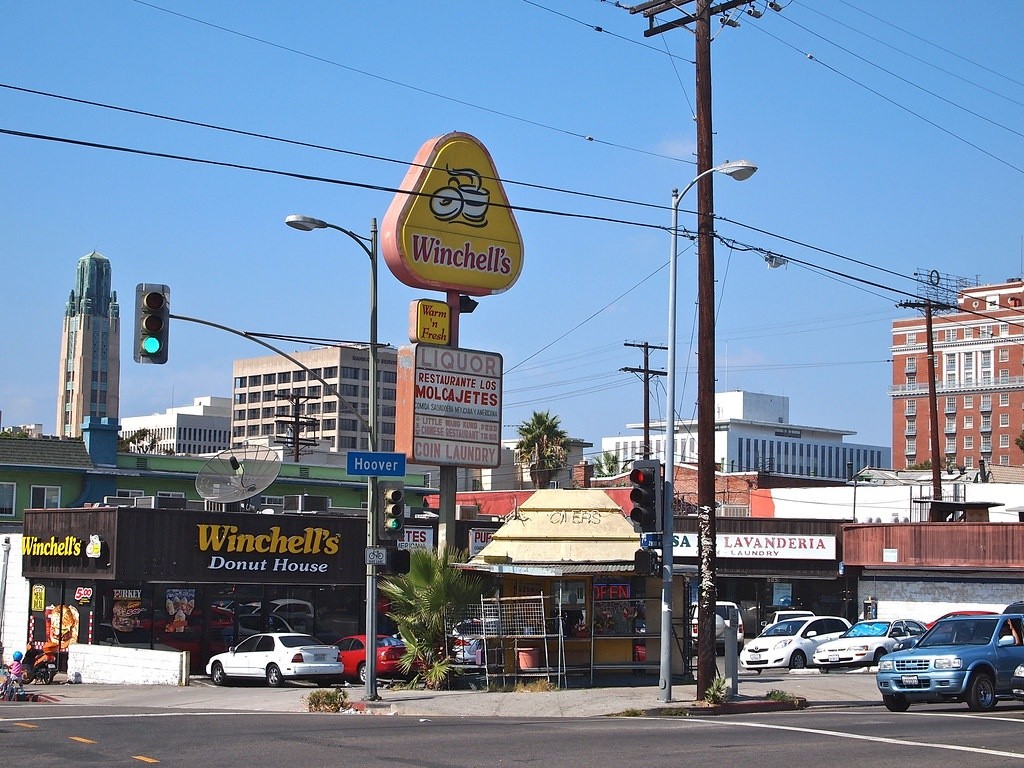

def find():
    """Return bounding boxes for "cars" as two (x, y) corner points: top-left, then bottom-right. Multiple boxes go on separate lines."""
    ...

(398, 637), (435, 660)
(740, 615), (853, 671)
(441, 635), (480, 664)
(812, 617), (930, 673)
(331, 634), (425, 685)
(213, 598), (315, 633)
(205, 632), (345, 689)
(924, 610), (1001, 631)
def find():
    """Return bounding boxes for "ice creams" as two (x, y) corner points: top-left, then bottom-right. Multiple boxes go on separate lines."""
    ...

(166, 597), (195, 632)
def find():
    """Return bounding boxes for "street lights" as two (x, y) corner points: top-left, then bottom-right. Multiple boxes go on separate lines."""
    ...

(658, 161), (760, 701)
(286, 214), (381, 699)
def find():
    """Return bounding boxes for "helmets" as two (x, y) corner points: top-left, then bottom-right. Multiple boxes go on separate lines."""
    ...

(13, 651), (23, 661)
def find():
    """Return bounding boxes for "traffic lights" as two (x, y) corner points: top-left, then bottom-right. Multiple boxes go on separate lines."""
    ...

(138, 289), (167, 357)
(389, 550), (411, 573)
(635, 551), (651, 574)
(384, 488), (405, 532)
(630, 467), (652, 527)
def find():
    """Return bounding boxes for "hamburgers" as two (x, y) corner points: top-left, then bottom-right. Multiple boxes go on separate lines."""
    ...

(50, 605), (74, 649)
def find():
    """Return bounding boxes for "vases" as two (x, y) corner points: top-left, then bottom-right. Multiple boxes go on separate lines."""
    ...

(513, 647), (541, 668)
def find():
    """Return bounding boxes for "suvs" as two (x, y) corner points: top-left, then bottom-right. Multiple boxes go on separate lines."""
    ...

(761, 610), (817, 638)
(689, 601), (745, 656)
(877, 613), (1024, 712)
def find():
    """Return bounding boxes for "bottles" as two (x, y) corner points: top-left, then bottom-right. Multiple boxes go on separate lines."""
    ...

(45, 603), (56, 643)
(166, 596), (195, 632)
(68, 605), (80, 644)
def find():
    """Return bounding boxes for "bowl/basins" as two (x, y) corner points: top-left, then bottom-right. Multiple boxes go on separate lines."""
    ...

(513, 648), (541, 668)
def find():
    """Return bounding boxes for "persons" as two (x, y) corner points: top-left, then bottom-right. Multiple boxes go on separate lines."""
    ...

(23, 642), (44, 684)
(5, 651), (26, 698)
(999, 619), (1021, 645)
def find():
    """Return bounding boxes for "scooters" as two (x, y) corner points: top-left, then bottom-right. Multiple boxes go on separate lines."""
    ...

(20, 639), (59, 685)
